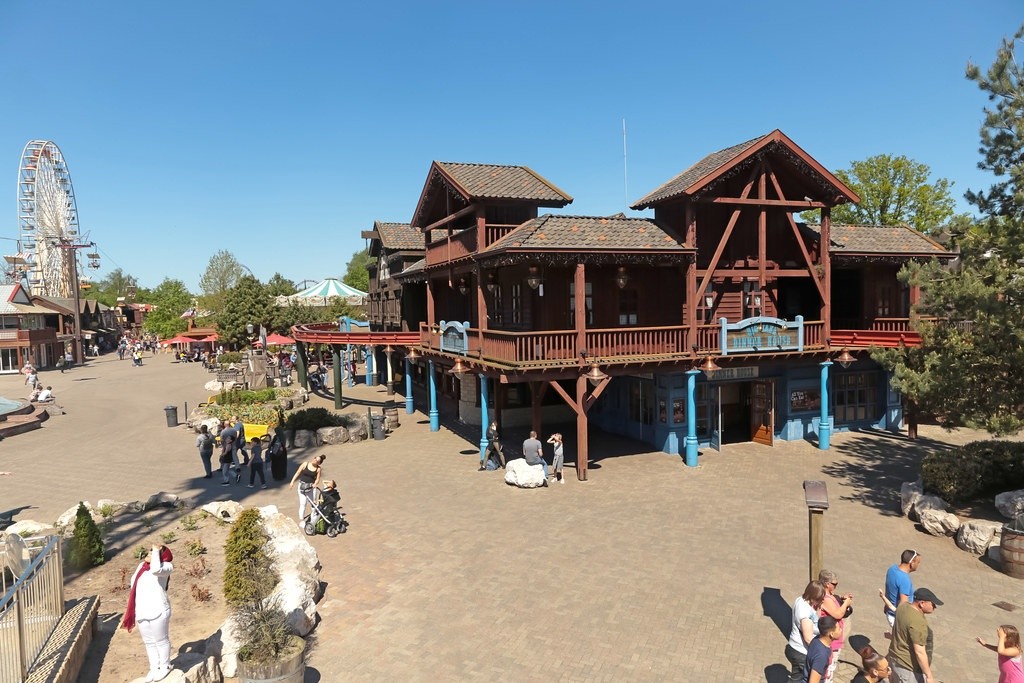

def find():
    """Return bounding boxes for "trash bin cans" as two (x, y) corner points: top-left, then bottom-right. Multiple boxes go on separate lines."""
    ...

(280, 373), (288, 387)
(371, 415), (387, 441)
(163, 406), (178, 427)
(284, 368), (292, 376)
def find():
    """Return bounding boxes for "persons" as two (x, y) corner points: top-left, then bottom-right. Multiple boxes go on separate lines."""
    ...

(117, 329), (161, 366)
(342, 359), (357, 385)
(197, 416), (287, 489)
(478, 420), (505, 471)
(547, 433), (565, 484)
(20, 361), (55, 405)
(176, 345), (225, 370)
(785, 550), (944, 683)
(131, 544), (174, 683)
(266, 350), (332, 390)
(975, 625), (1024, 683)
(522, 431), (549, 487)
(58, 338), (113, 373)
(290, 455), (336, 529)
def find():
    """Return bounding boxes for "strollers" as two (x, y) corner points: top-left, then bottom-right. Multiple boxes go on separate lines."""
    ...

(303, 486), (346, 538)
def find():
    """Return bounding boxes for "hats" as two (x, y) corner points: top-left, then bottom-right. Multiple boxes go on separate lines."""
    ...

(913, 587), (943, 605)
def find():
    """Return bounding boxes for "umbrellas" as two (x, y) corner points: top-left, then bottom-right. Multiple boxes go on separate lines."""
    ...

(252, 332), (297, 354)
(160, 335), (220, 353)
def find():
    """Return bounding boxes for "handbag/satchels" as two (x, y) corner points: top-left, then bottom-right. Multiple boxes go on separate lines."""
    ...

(832, 594), (852, 618)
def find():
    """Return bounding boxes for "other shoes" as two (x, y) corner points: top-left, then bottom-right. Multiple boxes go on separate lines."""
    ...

(235, 475), (240, 483)
(261, 484), (267, 488)
(204, 472), (212, 477)
(247, 484), (255, 488)
(154, 665), (173, 682)
(235, 468), (240, 472)
(145, 665), (159, 683)
(221, 482), (230, 487)
(502, 464), (505, 469)
(551, 477), (558, 482)
(299, 521), (306, 528)
(561, 479), (565, 484)
(242, 459), (250, 465)
(544, 478), (548, 487)
(217, 468), (223, 472)
(478, 466), (486, 471)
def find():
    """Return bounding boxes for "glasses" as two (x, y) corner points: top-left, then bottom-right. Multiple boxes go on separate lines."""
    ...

(910, 549), (918, 563)
(150, 548), (152, 551)
(830, 581), (838, 587)
(877, 665), (891, 673)
(314, 457), (319, 463)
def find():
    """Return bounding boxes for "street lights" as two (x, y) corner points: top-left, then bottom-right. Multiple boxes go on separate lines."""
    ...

(246, 321), (254, 356)
